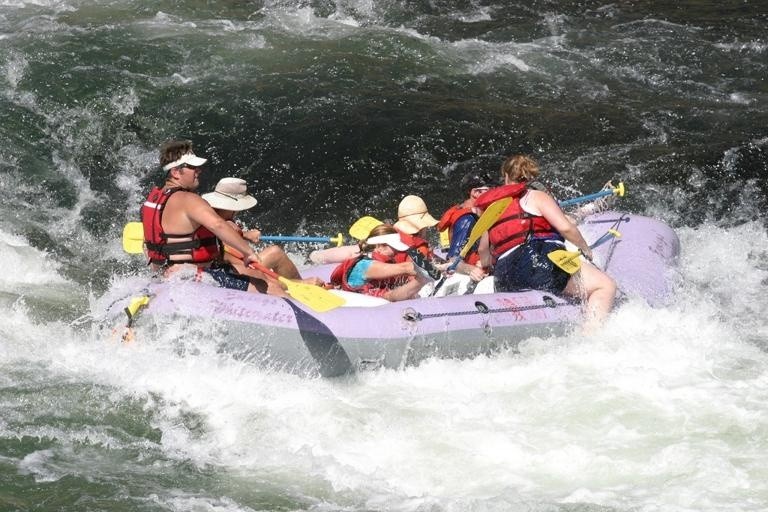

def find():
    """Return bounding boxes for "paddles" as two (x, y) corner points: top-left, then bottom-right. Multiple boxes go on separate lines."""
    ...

(429, 196), (512, 298)
(222, 244), (345, 315)
(122, 222), (343, 254)
(547, 229), (620, 275)
(349, 217), (384, 239)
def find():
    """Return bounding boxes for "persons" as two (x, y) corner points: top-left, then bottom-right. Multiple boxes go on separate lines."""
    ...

(436, 170), (617, 283)
(141, 139), (325, 300)
(329, 222), (424, 302)
(392, 193), (443, 279)
(471, 153), (617, 329)
(198, 176), (325, 288)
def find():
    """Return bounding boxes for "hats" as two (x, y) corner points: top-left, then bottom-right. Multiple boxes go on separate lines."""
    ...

(393, 195), (441, 234)
(462, 170), (499, 191)
(202, 178), (257, 211)
(163, 149), (208, 171)
(366, 233), (410, 252)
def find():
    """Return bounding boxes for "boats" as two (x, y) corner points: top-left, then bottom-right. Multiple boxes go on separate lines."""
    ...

(89, 208), (680, 381)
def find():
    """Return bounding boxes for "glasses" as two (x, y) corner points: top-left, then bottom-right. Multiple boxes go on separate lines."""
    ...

(175, 163), (196, 169)
